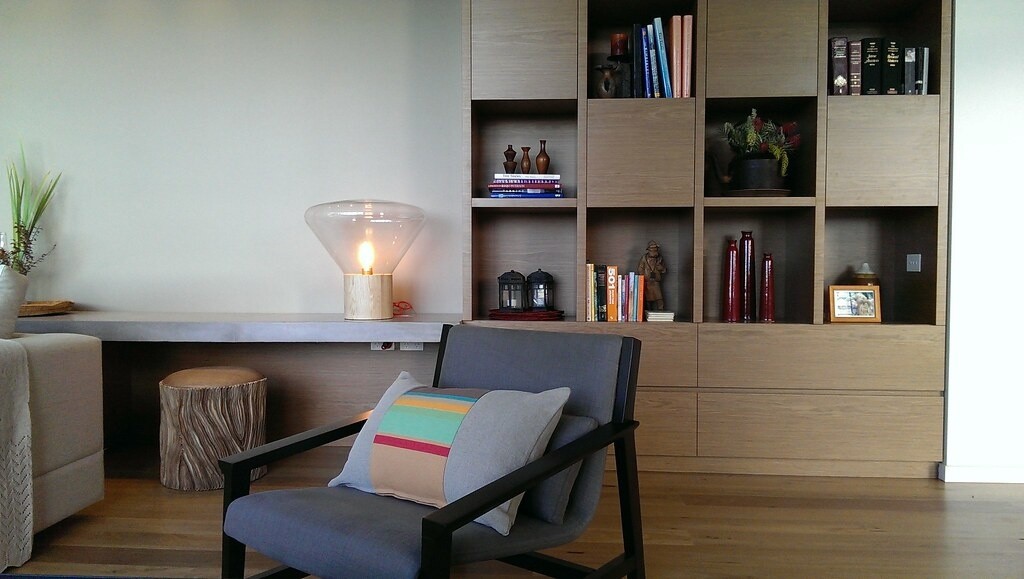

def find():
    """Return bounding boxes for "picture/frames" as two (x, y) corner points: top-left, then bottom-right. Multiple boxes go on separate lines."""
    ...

(827, 285), (882, 323)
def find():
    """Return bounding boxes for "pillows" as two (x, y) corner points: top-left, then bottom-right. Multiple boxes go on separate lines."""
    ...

(519, 414), (595, 525)
(0, 264), (29, 338)
(327, 371), (571, 536)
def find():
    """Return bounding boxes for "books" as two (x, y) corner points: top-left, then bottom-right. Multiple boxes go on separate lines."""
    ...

(630, 15), (692, 99)
(829, 36), (929, 95)
(586, 260), (675, 321)
(488, 172), (564, 198)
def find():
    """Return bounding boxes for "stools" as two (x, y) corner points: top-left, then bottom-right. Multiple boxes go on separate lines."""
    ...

(159, 366), (270, 491)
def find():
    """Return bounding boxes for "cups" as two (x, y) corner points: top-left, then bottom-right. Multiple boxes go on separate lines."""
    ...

(611, 34), (628, 55)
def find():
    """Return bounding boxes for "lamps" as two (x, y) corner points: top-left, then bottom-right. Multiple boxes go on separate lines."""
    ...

(305, 199), (426, 320)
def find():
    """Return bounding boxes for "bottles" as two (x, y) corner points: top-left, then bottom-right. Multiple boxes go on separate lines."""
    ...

(521, 147), (530, 173)
(536, 140), (550, 174)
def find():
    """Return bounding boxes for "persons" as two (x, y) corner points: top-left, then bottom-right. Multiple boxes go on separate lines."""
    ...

(637, 241), (667, 310)
(905, 50), (914, 62)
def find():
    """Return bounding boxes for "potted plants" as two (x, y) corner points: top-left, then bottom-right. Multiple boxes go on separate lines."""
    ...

(0, 143), (75, 316)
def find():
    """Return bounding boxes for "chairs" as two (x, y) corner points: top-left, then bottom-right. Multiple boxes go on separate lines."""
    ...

(219, 322), (645, 579)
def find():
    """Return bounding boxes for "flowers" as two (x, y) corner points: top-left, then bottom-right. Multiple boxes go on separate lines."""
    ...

(717, 110), (803, 177)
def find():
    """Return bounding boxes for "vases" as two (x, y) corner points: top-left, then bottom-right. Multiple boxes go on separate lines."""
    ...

(708, 150), (791, 197)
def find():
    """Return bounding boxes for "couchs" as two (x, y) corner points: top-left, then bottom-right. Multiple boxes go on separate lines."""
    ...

(0, 333), (103, 574)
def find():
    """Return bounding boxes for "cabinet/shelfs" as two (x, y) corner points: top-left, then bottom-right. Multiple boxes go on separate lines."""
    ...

(463, 0), (954, 484)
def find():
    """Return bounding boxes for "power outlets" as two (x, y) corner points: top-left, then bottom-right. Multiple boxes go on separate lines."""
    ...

(399, 342), (423, 351)
(370, 342), (395, 350)
